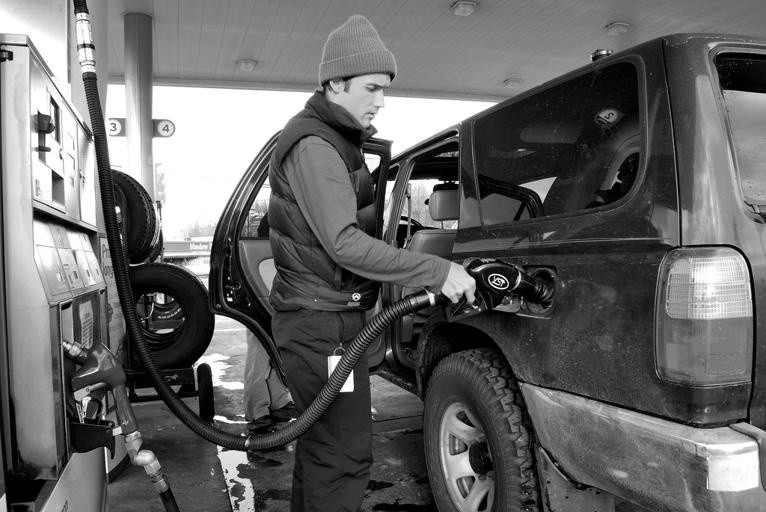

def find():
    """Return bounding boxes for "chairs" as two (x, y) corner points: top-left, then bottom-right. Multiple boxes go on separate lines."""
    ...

(403, 190), (464, 311)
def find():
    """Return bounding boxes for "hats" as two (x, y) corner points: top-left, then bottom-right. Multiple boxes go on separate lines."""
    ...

(319, 15), (396, 85)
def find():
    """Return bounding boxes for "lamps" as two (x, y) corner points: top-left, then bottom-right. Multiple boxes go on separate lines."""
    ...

(233, 58), (258, 74)
(605, 21), (631, 38)
(449, 0), (481, 18)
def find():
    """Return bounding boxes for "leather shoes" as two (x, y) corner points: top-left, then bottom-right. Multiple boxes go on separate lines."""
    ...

(281, 401), (297, 410)
(254, 415), (276, 427)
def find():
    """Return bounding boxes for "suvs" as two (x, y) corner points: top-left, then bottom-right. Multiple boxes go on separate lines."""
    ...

(191, 31), (766, 512)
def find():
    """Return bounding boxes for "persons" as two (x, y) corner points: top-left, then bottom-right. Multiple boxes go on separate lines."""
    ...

(243, 209), (299, 427)
(269, 15), (477, 510)
